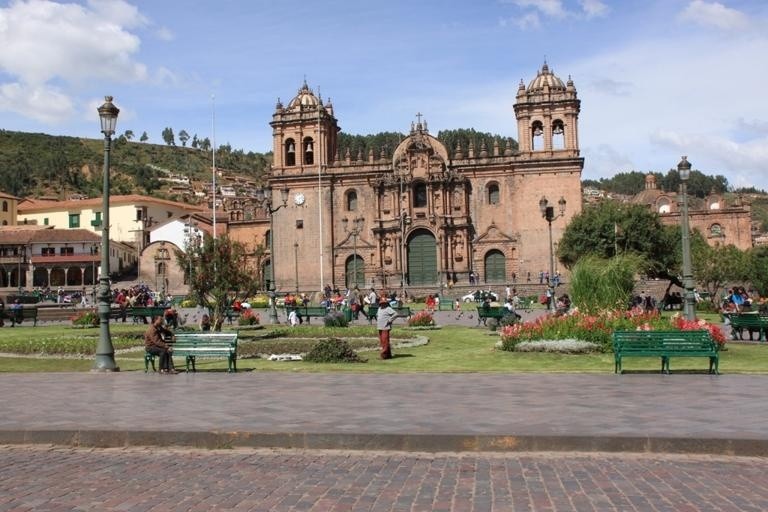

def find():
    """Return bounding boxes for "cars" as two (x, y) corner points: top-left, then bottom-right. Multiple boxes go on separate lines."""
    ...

(462, 290), (499, 304)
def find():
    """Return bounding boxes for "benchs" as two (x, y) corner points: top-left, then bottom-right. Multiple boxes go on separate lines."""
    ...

(474, 305), (522, 328)
(143, 329), (239, 373)
(0, 307), (38, 327)
(613, 329), (721, 376)
(131, 306), (172, 326)
(285, 305), (414, 326)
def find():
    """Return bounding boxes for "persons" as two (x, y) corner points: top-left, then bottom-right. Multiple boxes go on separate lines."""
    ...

(241, 300), (251, 309)
(640, 290), (647, 305)
(441, 281), (447, 288)
(8, 298), (24, 327)
(376, 296), (399, 360)
(144, 315), (180, 375)
(283, 284), (441, 327)
(455, 270), (571, 320)
(199, 313), (211, 331)
(661, 285), (768, 325)
(447, 279), (454, 289)
(21, 281), (178, 330)
(0, 298), (5, 327)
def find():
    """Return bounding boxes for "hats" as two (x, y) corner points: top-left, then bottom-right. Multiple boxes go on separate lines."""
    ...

(379, 297), (388, 304)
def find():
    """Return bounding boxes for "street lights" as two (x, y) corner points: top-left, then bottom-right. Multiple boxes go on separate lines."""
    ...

(91, 95), (119, 373)
(342, 215), (364, 288)
(18, 247), (22, 294)
(294, 242), (298, 296)
(677, 156), (697, 323)
(257, 184), (290, 325)
(539, 196), (567, 314)
(184, 220), (198, 295)
